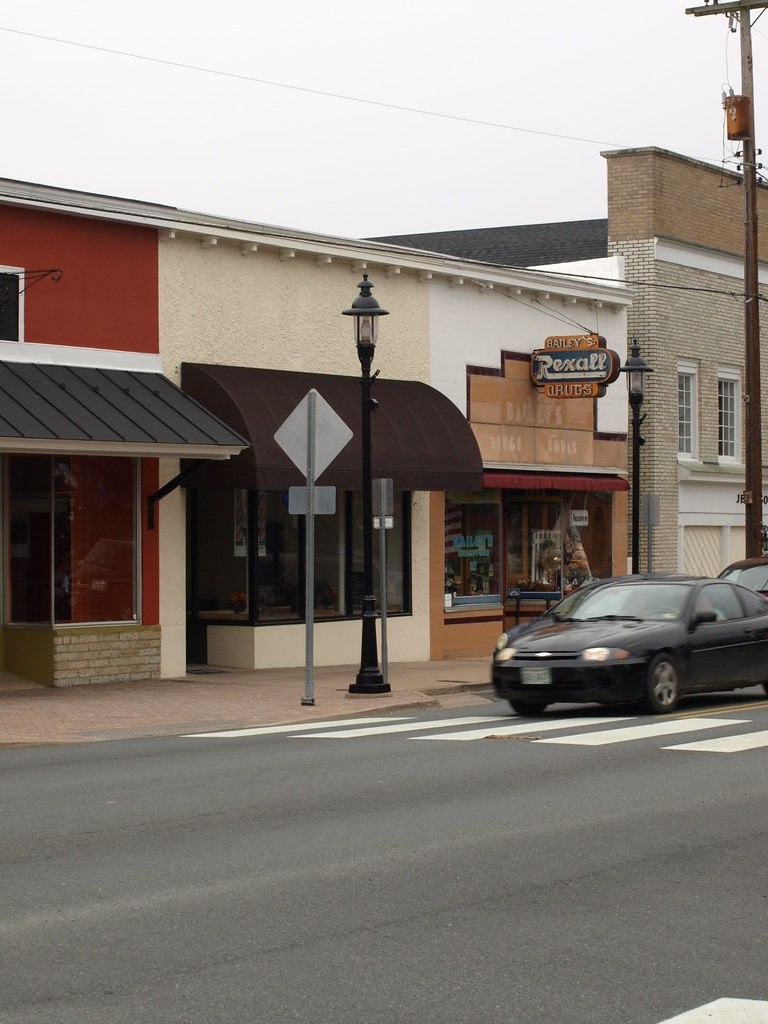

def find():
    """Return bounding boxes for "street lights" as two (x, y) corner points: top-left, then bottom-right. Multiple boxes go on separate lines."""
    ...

(341, 273), (392, 694)
(618, 339), (655, 575)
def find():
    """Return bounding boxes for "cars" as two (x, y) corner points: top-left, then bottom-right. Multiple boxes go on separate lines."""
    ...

(717, 557), (768, 601)
(491, 572), (691, 700)
(280, 554), (400, 612)
(61, 536), (137, 620)
(494, 575), (768, 715)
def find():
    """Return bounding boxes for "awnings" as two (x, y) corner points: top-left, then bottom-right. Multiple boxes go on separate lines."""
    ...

(483, 473), (631, 491)
(181, 362), (484, 491)
(0, 360), (251, 501)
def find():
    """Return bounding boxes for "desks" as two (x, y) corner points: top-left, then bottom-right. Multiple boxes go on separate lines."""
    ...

(507, 591), (561, 627)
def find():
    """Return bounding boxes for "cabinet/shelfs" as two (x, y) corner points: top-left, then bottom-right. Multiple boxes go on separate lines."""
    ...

(465, 506), (528, 595)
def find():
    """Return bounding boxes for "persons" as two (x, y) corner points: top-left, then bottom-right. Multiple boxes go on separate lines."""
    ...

(695, 590), (725, 621)
